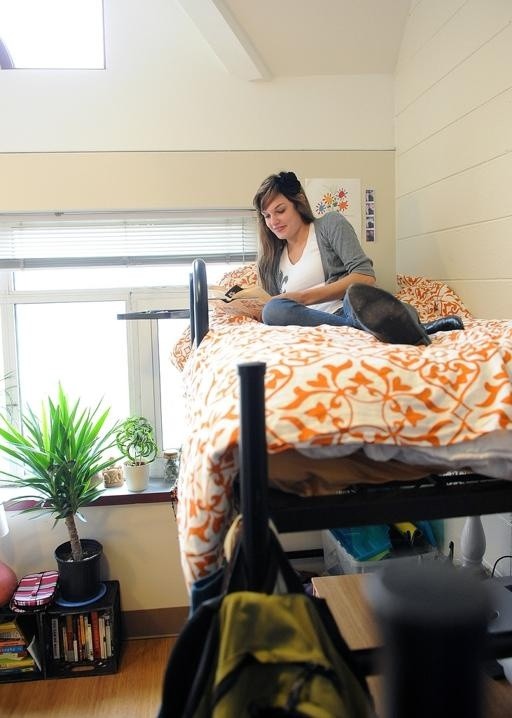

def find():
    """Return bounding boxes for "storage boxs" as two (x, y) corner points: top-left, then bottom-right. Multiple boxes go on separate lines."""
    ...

(322, 519), (441, 576)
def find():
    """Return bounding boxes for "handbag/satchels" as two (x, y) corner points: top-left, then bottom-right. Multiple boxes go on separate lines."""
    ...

(8, 569), (61, 614)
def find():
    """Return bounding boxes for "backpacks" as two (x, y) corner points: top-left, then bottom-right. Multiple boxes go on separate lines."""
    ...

(157, 527), (377, 718)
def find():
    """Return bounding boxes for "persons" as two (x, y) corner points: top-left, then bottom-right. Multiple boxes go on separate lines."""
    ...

(253, 171), (432, 347)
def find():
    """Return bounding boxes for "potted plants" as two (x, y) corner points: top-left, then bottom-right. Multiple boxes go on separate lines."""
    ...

(0, 377), (159, 602)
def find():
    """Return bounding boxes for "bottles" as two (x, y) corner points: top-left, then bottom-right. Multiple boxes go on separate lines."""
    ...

(163, 450), (179, 487)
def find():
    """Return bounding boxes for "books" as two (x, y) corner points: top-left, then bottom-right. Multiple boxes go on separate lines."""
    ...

(51, 611), (112, 662)
(0, 622), (42, 672)
(207, 285), (271, 303)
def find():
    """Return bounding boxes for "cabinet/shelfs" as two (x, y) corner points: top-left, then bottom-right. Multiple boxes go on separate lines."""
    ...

(1, 581), (125, 684)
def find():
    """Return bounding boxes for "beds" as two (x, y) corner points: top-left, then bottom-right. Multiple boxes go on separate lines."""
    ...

(191, 260), (509, 534)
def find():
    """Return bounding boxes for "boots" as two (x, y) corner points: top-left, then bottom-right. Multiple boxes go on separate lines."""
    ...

(347, 284), (464, 346)
